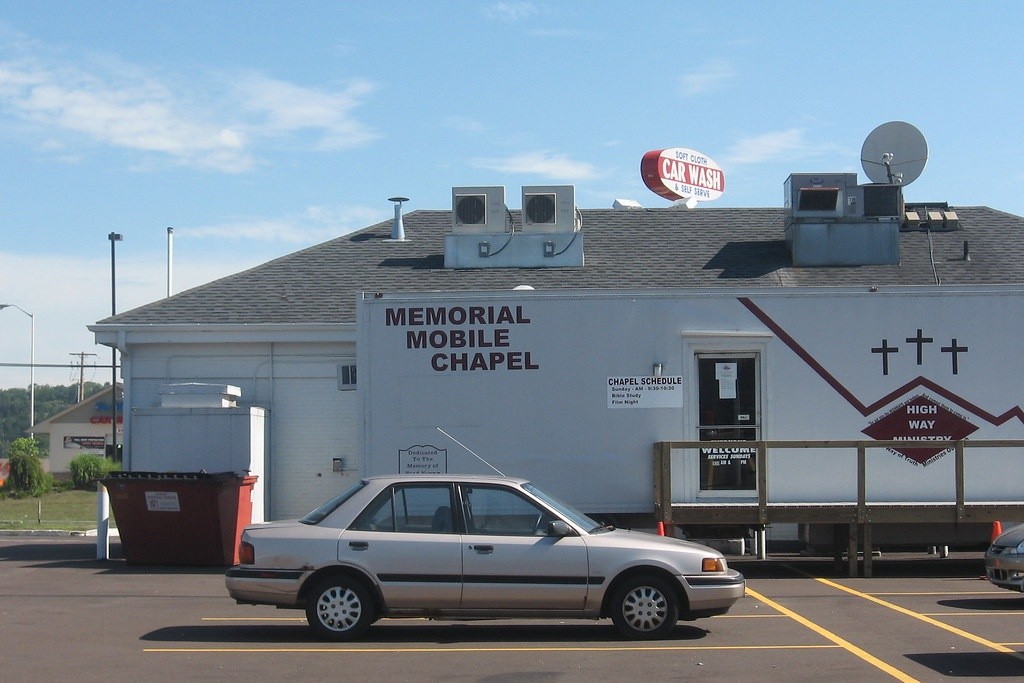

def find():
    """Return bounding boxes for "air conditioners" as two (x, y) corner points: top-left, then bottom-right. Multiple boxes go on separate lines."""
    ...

(521, 185), (576, 232)
(337, 363), (357, 391)
(452, 186), (506, 233)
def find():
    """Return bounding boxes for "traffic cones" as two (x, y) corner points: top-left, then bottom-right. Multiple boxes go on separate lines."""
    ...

(990, 521), (1002, 543)
(657, 521), (664, 537)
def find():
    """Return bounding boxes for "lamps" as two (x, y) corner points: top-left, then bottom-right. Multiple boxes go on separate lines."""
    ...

(654, 363), (663, 378)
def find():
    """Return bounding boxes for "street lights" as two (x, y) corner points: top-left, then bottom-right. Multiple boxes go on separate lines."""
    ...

(0, 302), (36, 443)
(108, 231), (124, 464)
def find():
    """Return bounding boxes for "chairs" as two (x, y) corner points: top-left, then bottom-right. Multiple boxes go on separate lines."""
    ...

(432, 506), (453, 531)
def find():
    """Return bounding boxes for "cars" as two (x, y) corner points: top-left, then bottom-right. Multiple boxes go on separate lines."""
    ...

(985, 523), (1024, 593)
(225, 472), (748, 642)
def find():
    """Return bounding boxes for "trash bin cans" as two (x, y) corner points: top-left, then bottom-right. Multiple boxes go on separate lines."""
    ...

(91, 470), (259, 567)
